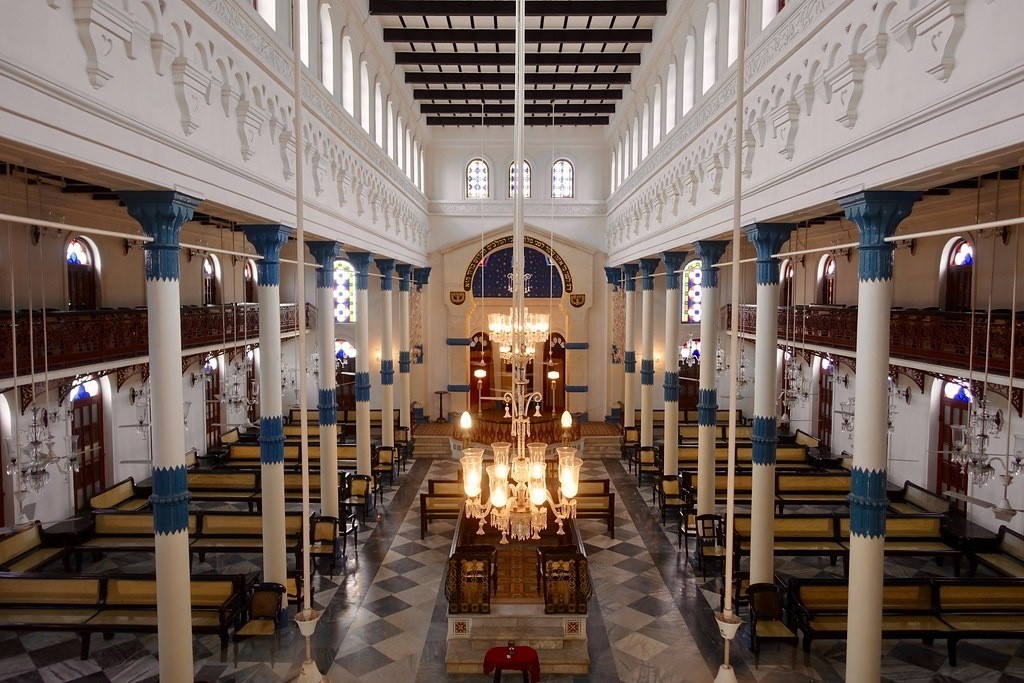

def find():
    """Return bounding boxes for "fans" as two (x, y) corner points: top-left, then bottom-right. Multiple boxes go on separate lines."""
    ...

(211, 226), (262, 430)
(776, 231), (825, 425)
(944, 160), (1023, 522)
(0, 156), (82, 536)
(720, 237), (754, 400)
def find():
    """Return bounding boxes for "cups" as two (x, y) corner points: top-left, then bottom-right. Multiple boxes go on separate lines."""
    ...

(508, 641), (515, 655)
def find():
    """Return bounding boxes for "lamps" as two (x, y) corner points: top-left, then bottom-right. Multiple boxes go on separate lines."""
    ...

(123, 229), (145, 255)
(897, 387), (911, 403)
(231, 244), (252, 267)
(189, 369), (208, 388)
(222, 221), (260, 414)
(726, 234), (752, 390)
(458, 0), (583, 544)
(786, 249), (807, 269)
(973, 207), (1011, 245)
(827, 373), (849, 391)
(896, 227), (916, 255)
(7, 159), (77, 492)
(127, 386), (151, 404)
(186, 239), (209, 260)
(828, 237), (852, 264)
(947, 172), (1023, 487)
(41, 404), (74, 427)
(30, 210), (65, 246)
(775, 221), (812, 409)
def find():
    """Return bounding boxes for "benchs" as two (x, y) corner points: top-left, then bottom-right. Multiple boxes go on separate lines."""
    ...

(0, 408), (401, 662)
(419, 479), (616, 541)
(635, 409), (1024, 667)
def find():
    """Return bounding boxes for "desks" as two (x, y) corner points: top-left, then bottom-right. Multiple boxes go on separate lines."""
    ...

(483, 646), (540, 683)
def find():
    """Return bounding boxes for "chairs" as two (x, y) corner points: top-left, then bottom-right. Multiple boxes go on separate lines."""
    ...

(394, 426), (408, 446)
(695, 514), (734, 579)
(370, 473), (383, 508)
(677, 511), (696, 555)
(719, 572), (749, 620)
(340, 474), (372, 525)
(394, 443), (406, 476)
(629, 444), (641, 475)
(372, 446), (396, 488)
(745, 583), (799, 672)
(638, 446), (662, 489)
(623, 427), (640, 461)
(286, 570), (315, 613)
(651, 474), (663, 510)
(301, 516), (339, 579)
(233, 583), (286, 670)
(338, 501), (359, 554)
(660, 475), (694, 527)
